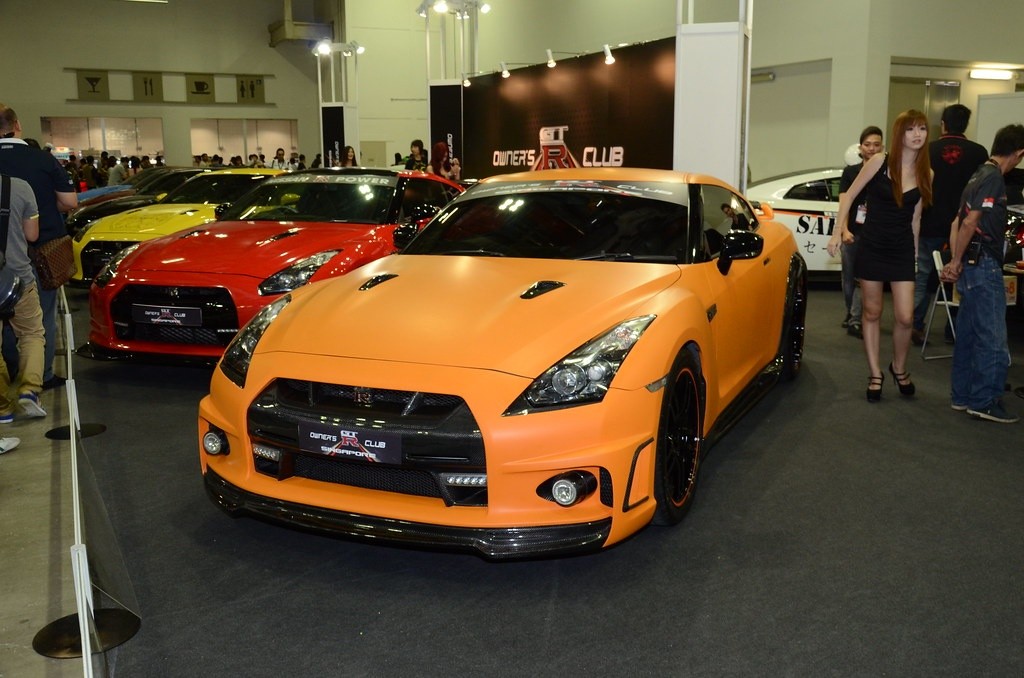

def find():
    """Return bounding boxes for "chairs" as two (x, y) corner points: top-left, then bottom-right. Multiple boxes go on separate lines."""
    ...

(918, 250), (1016, 366)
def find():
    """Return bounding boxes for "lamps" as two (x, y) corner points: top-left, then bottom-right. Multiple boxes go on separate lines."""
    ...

(545, 48), (580, 69)
(603, 42), (634, 65)
(969, 69), (1020, 81)
(311, 36), (333, 58)
(462, 70), (494, 88)
(341, 40), (366, 57)
(416, 0), (492, 21)
(499, 61), (536, 79)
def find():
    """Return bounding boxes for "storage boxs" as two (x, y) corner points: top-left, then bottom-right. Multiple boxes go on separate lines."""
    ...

(951, 276), (1017, 303)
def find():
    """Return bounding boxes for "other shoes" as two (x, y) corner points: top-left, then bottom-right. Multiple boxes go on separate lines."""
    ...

(42, 375), (67, 390)
(842, 313), (863, 339)
(910, 329), (931, 346)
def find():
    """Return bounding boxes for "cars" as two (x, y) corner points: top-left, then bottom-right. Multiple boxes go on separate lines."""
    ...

(68, 167), (303, 282)
(944, 202), (1024, 316)
(65, 164), (210, 232)
(744, 168), (868, 273)
(74, 168), (501, 362)
(198, 167), (806, 554)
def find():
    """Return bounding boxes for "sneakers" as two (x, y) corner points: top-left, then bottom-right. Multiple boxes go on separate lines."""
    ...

(0, 413), (13, 424)
(951, 398), (970, 410)
(19, 388), (47, 417)
(0, 434), (21, 455)
(966, 399), (1020, 422)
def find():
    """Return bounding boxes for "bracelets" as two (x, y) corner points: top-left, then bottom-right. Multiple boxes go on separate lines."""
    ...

(448, 172), (451, 176)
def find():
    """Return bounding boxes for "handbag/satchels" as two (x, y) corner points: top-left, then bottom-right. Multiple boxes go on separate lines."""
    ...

(848, 153), (891, 233)
(33, 235), (79, 291)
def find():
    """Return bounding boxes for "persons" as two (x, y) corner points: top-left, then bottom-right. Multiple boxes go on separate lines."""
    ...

(338, 146), (357, 167)
(0, 101), (78, 423)
(61, 152), (166, 192)
(405, 140), (428, 171)
(291, 153), (322, 170)
(721, 203), (749, 230)
(264, 148), (288, 169)
(426, 142), (460, 180)
(826, 109), (934, 402)
(839, 126), (884, 338)
(940, 125), (1024, 422)
(195, 154), (265, 168)
(912, 105), (989, 345)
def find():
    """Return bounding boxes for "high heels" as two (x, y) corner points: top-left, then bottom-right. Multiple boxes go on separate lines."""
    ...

(889, 361), (916, 397)
(867, 372), (885, 402)
